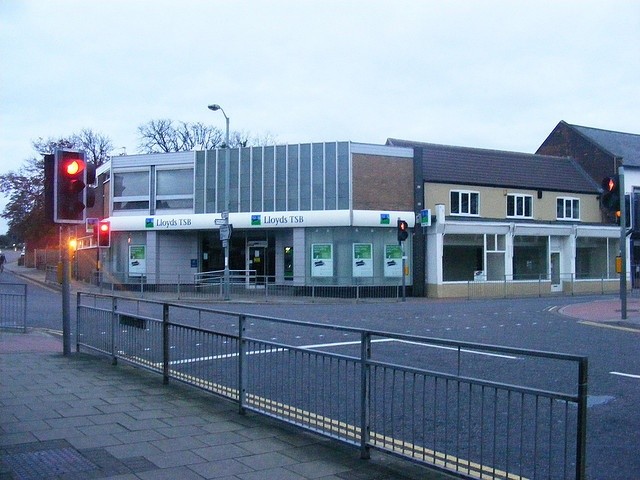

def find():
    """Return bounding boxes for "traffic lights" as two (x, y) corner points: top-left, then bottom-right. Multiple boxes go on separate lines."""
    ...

(97, 220), (110, 248)
(600, 174), (619, 210)
(53, 148), (87, 223)
(397, 219), (408, 241)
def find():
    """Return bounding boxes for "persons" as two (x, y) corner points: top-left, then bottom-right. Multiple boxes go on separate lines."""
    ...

(0, 251), (6, 273)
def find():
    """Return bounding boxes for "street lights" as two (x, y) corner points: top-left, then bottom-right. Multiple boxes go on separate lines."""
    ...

(207, 103), (230, 301)
